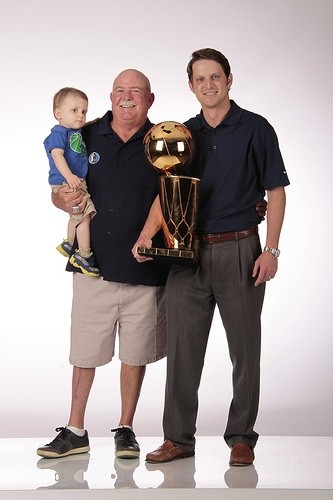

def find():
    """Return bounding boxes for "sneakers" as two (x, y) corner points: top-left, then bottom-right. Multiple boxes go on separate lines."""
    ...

(55, 239), (72, 257)
(111, 424), (141, 458)
(69, 251), (100, 277)
(37, 426), (89, 457)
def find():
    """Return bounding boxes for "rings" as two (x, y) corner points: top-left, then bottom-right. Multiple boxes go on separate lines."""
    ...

(269, 276), (272, 279)
(72, 205), (80, 214)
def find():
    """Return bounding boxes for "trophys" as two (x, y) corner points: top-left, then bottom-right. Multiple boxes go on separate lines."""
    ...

(137, 121), (202, 265)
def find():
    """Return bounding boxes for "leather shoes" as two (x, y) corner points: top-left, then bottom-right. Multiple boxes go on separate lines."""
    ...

(145, 440), (195, 463)
(230, 443), (255, 466)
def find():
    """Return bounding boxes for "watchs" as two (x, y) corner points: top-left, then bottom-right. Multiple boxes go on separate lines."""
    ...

(264, 247), (280, 258)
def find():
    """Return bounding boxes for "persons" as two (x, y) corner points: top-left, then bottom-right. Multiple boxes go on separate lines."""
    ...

(36, 69), (167, 459)
(43, 87), (101, 277)
(131, 48), (290, 466)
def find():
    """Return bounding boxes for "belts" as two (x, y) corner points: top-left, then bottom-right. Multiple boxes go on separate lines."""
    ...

(197, 227), (258, 245)
